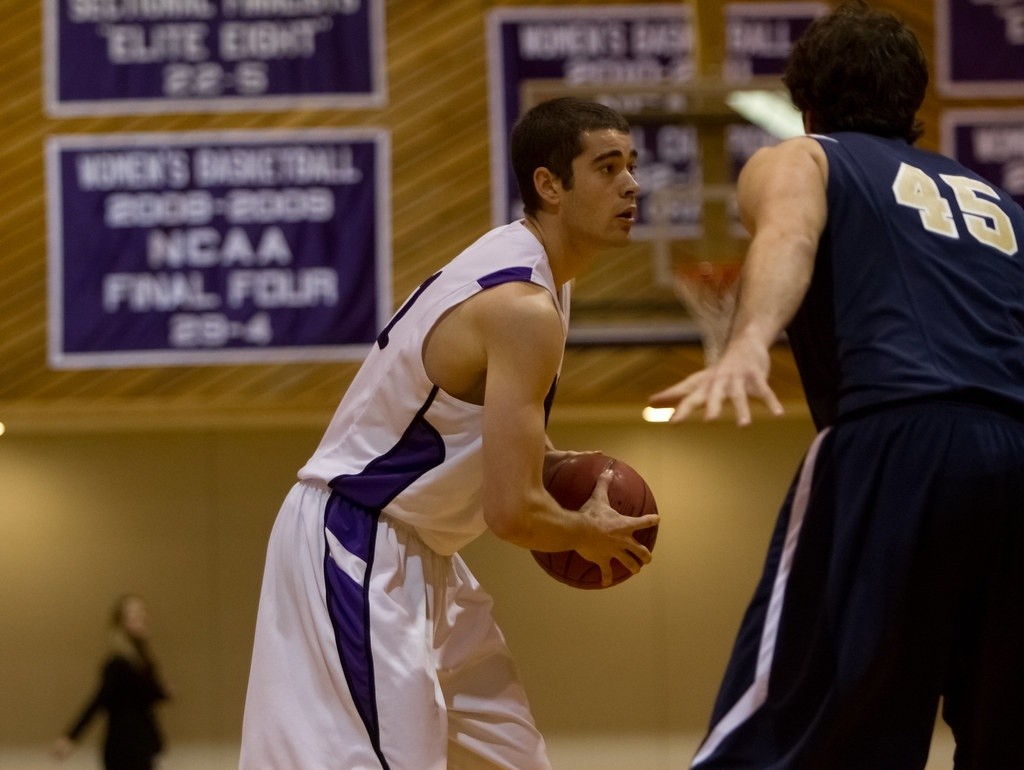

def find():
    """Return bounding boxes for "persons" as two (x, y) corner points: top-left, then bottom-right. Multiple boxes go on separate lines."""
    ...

(649, 9), (1024, 770)
(58, 596), (181, 770)
(234, 96), (661, 770)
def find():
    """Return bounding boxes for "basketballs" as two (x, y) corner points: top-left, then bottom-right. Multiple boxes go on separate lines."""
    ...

(526, 453), (658, 590)
